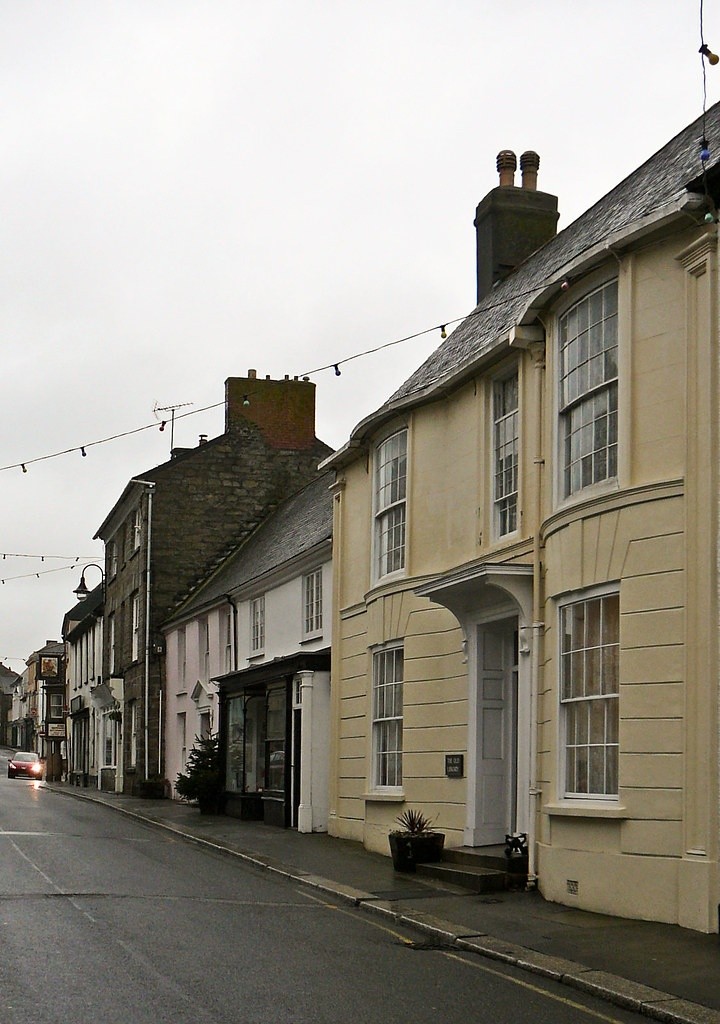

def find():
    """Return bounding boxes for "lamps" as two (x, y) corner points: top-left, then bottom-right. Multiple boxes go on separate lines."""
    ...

(138, 774), (165, 799)
(73, 564), (107, 605)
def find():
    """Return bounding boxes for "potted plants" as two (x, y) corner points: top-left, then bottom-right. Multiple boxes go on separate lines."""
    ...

(173, 728), (223, 815)
(389, 807), (446, 873)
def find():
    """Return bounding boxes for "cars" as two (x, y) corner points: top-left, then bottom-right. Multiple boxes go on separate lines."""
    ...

(270, 750), (284, 765)
(8, 752), (44, 780)
